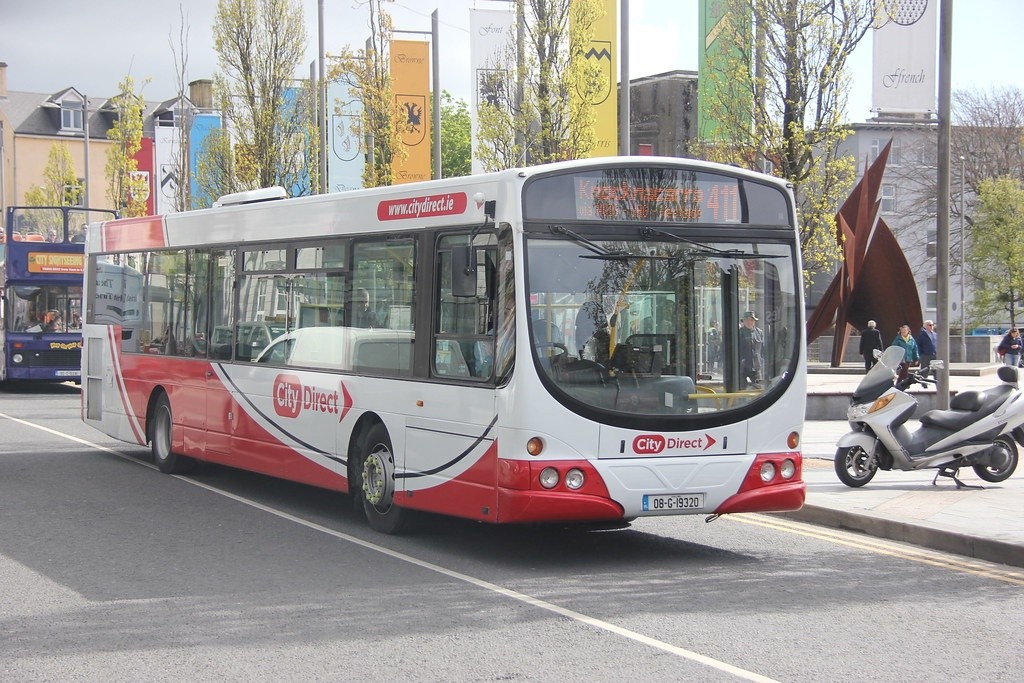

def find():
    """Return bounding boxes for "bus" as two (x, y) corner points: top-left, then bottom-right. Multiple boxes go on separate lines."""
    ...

(1, 204), (122, 390)
(80, 156), (806, 536)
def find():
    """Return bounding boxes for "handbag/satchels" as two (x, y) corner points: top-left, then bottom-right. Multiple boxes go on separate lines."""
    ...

(998, 346), (1007, 355)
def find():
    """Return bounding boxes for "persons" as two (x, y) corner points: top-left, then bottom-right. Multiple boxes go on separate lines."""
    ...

(999, 327), (1024, 366)
(474, 297), (542, 377)
(336, 287), (382, 328)
(892, 325), (918, 388)
(859, 320), (883, 371)
(71, 224), (87, 242)
(71, 312), (82, 329)
(739, 311), (763, 383)
(46, 230), (61, 243)
(915, 320), (937, 388)
(709, 321), (720, 369)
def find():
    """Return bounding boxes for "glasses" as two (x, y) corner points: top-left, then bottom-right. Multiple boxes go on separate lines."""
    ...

(929, 324), (934, 327)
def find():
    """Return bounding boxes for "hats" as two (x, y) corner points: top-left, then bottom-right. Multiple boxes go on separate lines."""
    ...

(741, 311), (759, 322)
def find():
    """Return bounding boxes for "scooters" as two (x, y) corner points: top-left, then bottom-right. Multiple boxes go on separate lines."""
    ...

(834, 319), (1024, 489)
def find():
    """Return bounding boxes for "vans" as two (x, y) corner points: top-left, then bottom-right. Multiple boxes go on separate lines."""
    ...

(250, 327), (473, 379)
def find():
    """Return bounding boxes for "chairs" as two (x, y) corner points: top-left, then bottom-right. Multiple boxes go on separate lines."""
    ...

(534, 320), (564, 356)
